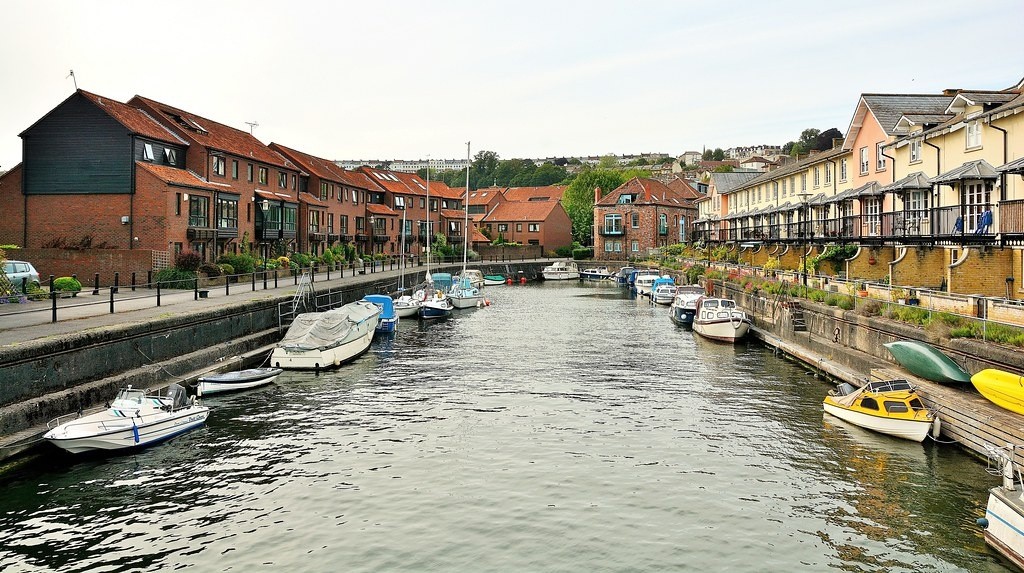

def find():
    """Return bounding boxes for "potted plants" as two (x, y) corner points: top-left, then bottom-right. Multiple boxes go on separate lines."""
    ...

(154, 240), (388, 289)
(812, 280), (920, 306)
(424, 232), (481, 263)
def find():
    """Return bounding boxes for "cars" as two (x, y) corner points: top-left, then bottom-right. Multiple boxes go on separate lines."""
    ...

(1, 260), (41, 293)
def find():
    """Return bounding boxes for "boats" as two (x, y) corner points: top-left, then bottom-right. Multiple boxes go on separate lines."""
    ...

(581, 266), (612, 279)
(393, 141), (506, 318)
(970, 369), (1024, 415)
(883, 340), (972, 383)
(197, 368), (284, 397)
(976, 443), (1024, 571)
(612, 266), (707, 326)
(361, 295), (399, 333)
(541, 261), (581, 280)
(270, 300), (383, 370)
(821, 379), (934, 442)
(692, 296), (751, 343)
(42, 384), (210, 454)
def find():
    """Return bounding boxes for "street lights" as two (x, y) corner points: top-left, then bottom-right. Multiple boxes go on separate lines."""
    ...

(257, 200), (273, 289)
(796, 189), (814, 283)
(415, 218), (420, 266)
(664, 222), (669, 261)
(368, 216), (375, 273)
(704, 212), (714, 265)
